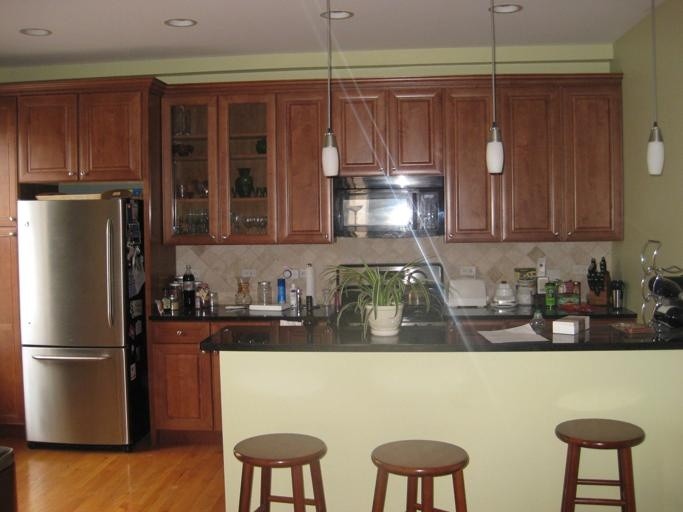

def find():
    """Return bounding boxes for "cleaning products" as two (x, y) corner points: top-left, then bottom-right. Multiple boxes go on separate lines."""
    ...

(290, 285), (297, 306)
(277, 279), (285, 305)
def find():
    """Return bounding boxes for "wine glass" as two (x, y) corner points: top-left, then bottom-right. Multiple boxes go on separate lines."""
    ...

(231, 212), (267, 238)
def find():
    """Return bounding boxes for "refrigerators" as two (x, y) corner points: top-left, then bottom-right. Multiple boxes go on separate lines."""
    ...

(16, 199), (151, 452)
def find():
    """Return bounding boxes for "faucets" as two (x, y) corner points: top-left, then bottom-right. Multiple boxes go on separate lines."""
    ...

(297, 288), (301, 315)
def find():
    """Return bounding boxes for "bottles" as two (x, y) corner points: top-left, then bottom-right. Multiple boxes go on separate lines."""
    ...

(528, 308), (545, 334)
(255, 262), (341, 312)
(608, 279), (624, 314)
(543, 281), (556, 316)
(154, 264), (219, 316)
(172, 207), (210, 237)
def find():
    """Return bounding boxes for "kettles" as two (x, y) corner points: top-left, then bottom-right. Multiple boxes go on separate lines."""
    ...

(515, 279), (538, 307)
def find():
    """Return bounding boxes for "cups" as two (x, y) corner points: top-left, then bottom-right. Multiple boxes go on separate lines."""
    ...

(178, 143), (192, 157)
(535, 256), (545, 276)
(231, 185), (266, 198)
(175, 180), (209, 200)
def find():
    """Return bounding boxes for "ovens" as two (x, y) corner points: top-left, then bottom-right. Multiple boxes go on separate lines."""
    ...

(334, 320), (448, 348)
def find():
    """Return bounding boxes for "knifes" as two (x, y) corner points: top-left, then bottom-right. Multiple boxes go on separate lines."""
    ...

(586, 256), (606, 296)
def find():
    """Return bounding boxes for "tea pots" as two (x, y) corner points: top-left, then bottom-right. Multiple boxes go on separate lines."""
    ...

(490, 280), (517, 309)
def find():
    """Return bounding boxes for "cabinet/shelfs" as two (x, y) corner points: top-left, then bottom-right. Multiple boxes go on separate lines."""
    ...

(148, 320), (280, 447)
(331, 77), (444, 176)
(0, 76), (176, 427)
(444, 73), (624, 243)
(162, 79), (334, 246)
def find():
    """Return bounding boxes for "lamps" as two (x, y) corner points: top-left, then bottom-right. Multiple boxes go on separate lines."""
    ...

(486, 1), (504, 174)
(322, 0), (339, 177)
(646, 0), (665, 176)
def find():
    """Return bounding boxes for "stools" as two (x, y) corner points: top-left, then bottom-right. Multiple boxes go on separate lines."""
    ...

(555, 419), (645, 512)
(371, 440), (469, 512)
(234, 434), (327, 512)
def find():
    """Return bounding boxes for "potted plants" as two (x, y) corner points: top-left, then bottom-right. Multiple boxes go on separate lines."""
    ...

(318, 254), (459, 338)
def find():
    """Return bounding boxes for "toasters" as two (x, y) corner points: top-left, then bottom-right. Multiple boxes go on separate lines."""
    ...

(446, 279), (488, 311)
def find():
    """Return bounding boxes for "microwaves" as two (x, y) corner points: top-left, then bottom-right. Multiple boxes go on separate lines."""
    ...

(333, 177), (443, 238)
